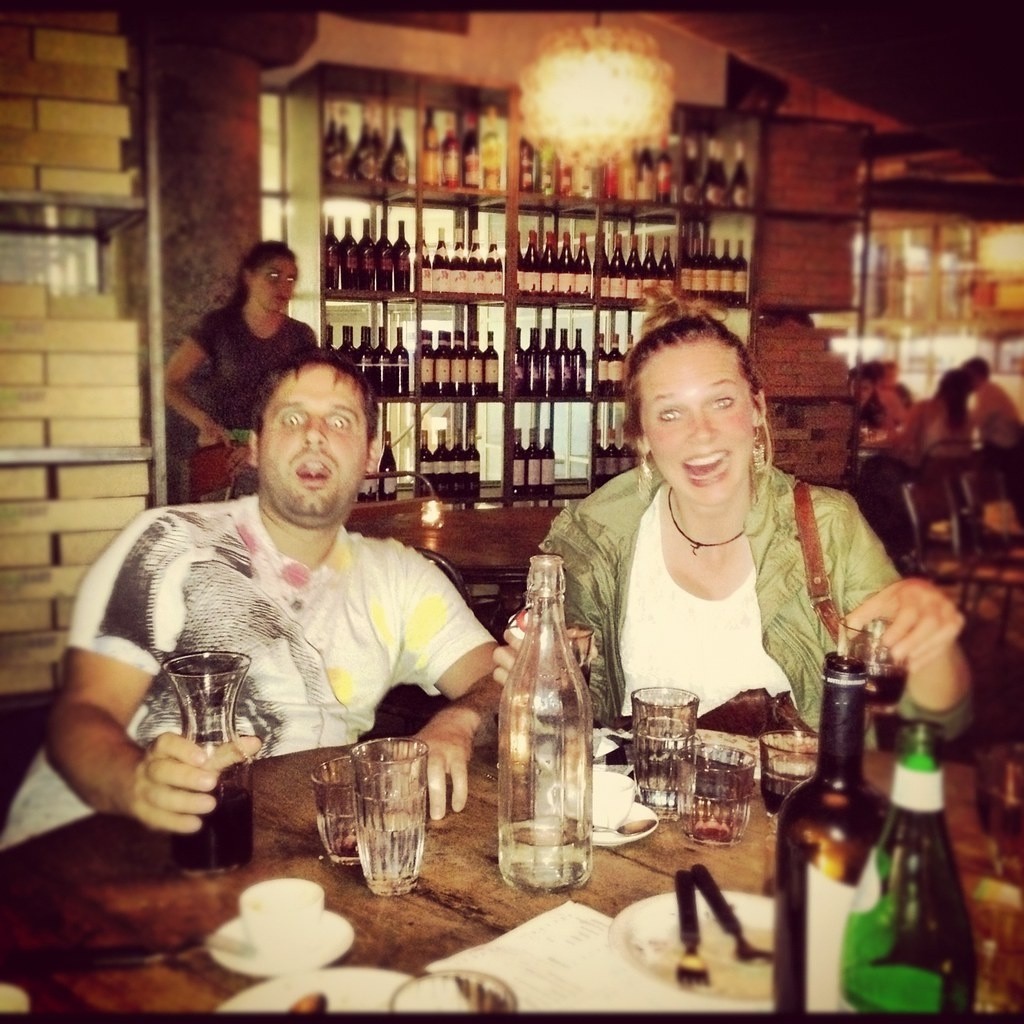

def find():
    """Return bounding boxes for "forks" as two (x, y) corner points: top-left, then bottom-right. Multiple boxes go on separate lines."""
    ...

(62, 935), (255, 970)
(675, 868), (709, 987)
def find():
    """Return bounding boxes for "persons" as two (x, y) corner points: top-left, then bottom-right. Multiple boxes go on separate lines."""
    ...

(846, 357), (1024, 459)
(163, 240), (318, 449)
(490, 310), (970, 743)
(0, 346), (503, 849)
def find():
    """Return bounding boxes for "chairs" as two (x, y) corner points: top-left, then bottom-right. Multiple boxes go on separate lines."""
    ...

(932, 439), (1024, 647)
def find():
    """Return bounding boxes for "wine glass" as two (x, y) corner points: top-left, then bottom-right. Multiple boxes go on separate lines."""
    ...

(971, 743), (1023, 963)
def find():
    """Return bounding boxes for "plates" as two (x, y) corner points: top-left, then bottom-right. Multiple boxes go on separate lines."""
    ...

(592, 802), (659, 846)
(609, 891), (775, 1012)
(217, 967), (471, 1013)
(206, 910), (354, 978)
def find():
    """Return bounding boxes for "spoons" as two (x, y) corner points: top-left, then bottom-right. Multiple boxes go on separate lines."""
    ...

(689, 864), (773, 962)
(595, 818), (657, 836)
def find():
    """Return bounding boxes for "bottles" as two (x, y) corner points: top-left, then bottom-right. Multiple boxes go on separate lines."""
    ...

(320, 325), (644, 501)
(839, 721), (977, 1013)
(775, 651), (889, 1014)
(322, 98), (749, 305)
(161, 650), (255, 872)
(497, 555), (593, 891)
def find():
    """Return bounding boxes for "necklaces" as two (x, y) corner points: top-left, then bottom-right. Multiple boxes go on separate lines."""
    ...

(668, 486), (745, 557)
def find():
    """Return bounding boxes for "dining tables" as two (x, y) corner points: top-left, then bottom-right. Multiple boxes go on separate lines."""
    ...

(0, 738), (1021, 1013)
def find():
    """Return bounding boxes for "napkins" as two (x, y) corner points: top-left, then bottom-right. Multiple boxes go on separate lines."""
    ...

(427, 897), (656, 1012)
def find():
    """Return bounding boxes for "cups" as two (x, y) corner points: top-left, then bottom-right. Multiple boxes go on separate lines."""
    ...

(757, 729), (820, 820)
(348, 736), (428, 898)
(632, 686), (700, 807)
(310, 756), (360, 867)
(552, 618), (595, 688)
(239, 879), (325, 951)
(388, 970), (517, 1012)
(547, 772), (636, 829)
(853, 618), (912, 712)
(675, 744), (756, 846)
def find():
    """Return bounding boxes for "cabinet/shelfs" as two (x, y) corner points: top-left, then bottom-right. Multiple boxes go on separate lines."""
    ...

(277, 75), (759, 512)
